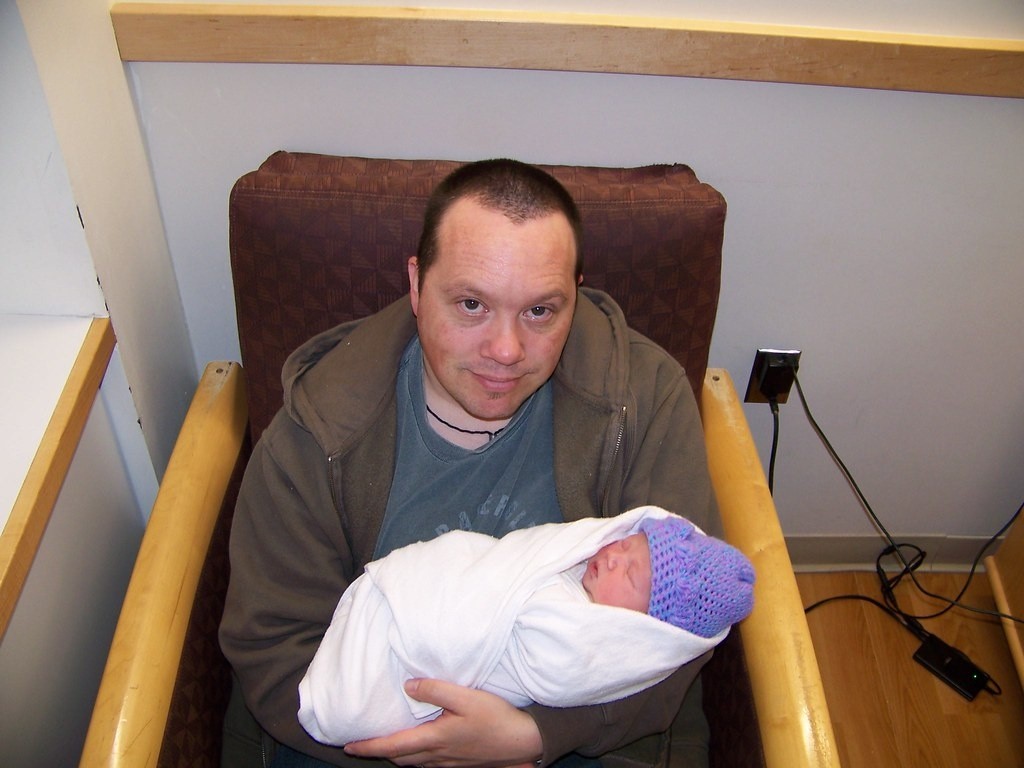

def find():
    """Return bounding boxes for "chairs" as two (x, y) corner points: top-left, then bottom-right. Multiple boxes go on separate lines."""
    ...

(77, 151), (846, 768)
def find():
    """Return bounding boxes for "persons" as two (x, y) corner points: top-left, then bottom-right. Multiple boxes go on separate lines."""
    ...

(296, 505), (754, 746)
(218, 158), (727, 768)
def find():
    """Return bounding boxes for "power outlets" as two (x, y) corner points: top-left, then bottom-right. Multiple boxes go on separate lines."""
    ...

(743, 348), (802, 405)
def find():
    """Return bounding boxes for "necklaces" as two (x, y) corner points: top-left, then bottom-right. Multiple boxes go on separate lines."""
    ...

(426, 405), (504, 440)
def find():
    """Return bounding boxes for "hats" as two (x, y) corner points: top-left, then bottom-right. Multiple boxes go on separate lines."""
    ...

(639, 515), (756, 638)
